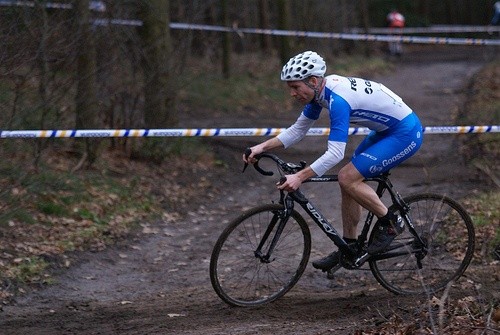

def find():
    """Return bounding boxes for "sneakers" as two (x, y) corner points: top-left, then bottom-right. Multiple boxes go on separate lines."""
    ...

(367, 215), (405, 254)
(312, 250), (340, 270)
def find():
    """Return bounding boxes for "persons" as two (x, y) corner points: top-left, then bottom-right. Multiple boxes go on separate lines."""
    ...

(242, 50), (422, 270)
(386, 10), (405, 29)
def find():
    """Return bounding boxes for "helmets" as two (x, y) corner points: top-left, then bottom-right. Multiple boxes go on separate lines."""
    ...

(281, 51), (328, 81)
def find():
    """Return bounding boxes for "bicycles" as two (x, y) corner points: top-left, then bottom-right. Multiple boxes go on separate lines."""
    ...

(209, 146), (477, 309)
